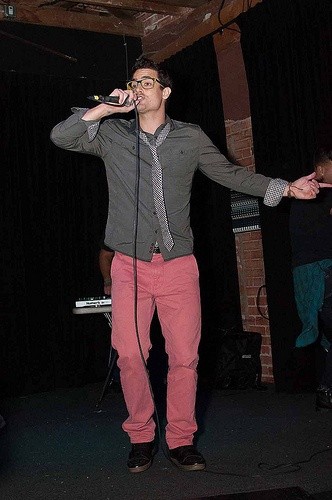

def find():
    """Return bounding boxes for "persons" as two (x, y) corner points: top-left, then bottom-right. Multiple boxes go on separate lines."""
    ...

(98, 245), (115, 296)
(287, 150), (332, 348)
(50, 58), (332, 472)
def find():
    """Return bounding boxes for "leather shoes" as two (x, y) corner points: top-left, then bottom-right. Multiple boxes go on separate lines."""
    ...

(169, 445), (205, 470)
(126, 437), (158, 473)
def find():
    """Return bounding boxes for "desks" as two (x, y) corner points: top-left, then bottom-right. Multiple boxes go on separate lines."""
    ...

(73, 306), (118, 414)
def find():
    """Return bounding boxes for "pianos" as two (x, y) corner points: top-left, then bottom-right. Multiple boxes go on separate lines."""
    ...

(72, 293), (158, 416)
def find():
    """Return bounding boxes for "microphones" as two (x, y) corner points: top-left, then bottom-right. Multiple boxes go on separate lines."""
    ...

(88, 95), (134, 104)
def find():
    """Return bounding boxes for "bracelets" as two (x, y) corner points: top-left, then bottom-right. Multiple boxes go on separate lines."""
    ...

(287, 181), (293, 198)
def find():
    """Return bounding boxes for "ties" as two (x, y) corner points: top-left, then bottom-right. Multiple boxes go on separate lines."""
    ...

(135, 126), (175, 252)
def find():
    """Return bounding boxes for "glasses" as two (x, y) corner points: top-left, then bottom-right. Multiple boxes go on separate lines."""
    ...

(124, 77), (166, 90)
